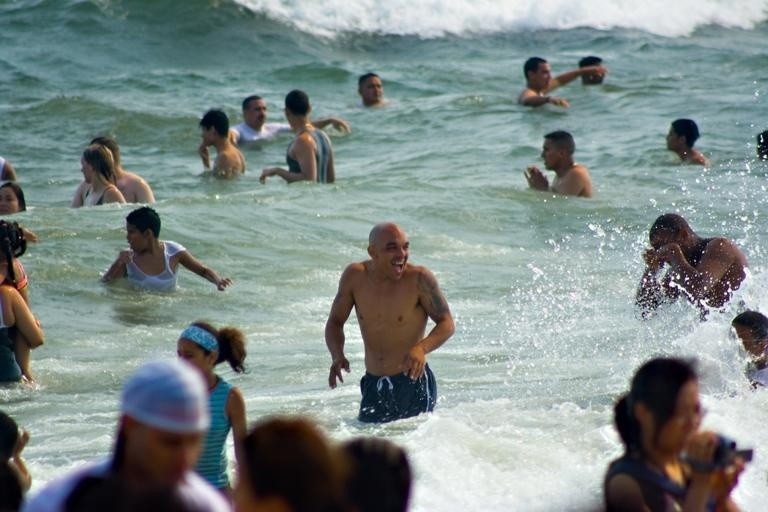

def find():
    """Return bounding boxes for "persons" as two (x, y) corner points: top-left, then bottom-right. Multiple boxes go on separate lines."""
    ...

(198, 109), (248, 183)
(334, 434), (412, 512)
(574, 54), (610, 87)
(232, 412), (340, 512)
(80, 145), (126, 208)
(355, 73), (387, 107)
(71, 135), (162, 206)
(259, 90), (335, 186)
(517, 57), (604, 111)
(0, 222), (38, 384)
(1, 413), (32, 512)
(99, 207), (235, 294)
(728, 309), (768, 388)
(753, 129), (768, 163)
(601, 356), (744, 512)
(0, 156), (24, 185)
(0, 240), (44, 385)
(0, 182), (28, 216)
(522, 130), (595, 201)
(177, 321), (251, 512)
(324, 221), (457, 423)
(25, 357), (231, 512)
(665, 117), (710, 169)
(199, 92), (357, 141)
(635, 213), (750, 318)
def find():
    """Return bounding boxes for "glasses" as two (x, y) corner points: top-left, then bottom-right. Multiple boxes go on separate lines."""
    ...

(669, 407), (707, 430)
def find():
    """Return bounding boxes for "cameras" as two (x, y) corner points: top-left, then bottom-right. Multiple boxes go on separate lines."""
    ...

(713, 435), (752, 467)
